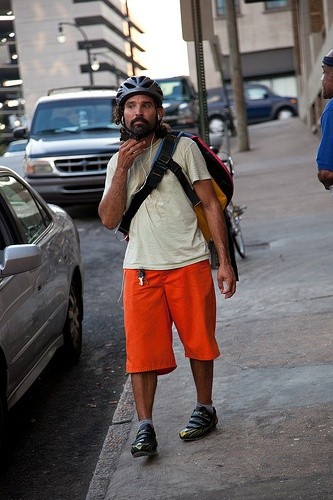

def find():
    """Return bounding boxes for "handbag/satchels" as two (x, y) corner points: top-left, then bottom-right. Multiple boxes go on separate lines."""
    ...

(156, 131), (235, 244)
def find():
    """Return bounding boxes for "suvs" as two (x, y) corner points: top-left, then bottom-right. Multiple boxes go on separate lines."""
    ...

(13, 86), (127, 207)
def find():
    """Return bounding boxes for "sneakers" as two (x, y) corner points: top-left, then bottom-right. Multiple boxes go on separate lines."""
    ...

(131, 424), (159, 458)
(179, 406), (219, 442)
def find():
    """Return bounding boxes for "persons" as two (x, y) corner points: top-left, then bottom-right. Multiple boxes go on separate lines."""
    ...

(316, 48), (333, 191)
(98, 75), (238, 459)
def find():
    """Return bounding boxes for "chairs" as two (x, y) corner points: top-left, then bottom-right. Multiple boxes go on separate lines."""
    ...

(51, 117), (71, 128)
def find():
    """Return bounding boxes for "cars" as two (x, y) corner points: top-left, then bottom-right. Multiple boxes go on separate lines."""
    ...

(0, 165), (85, 446)
(152, 75), (201, 141)
(0, 139), (29, 179)
(195, 82), (300, 133)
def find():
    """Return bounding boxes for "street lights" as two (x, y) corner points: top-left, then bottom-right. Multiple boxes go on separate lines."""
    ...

(56, 20), (94, 88)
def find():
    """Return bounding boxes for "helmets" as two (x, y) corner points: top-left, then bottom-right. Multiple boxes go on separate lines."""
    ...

(115, 76), (164, 104)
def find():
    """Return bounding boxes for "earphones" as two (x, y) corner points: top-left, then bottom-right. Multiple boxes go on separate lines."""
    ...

(158, 112), (160, 120)
(121, 115), (123, 123)
